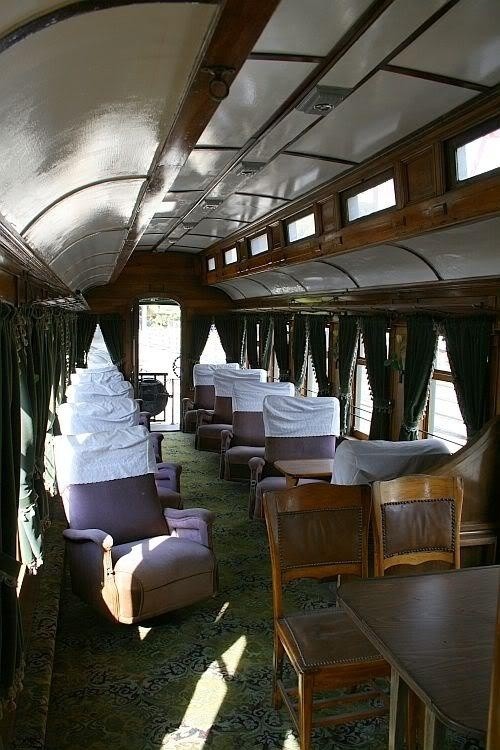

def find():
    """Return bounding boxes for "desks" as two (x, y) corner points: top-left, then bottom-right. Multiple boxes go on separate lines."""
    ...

(336, 564), (500, 740)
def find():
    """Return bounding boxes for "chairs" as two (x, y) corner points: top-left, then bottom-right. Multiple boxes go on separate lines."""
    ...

(263, 480), (388, 747)
(329, 434), (452, 489)
(370, 475), (465, 575)
(54, 426), (219, 632)
(51, 363), (187, 514)
(180, 364), (344, 519)
